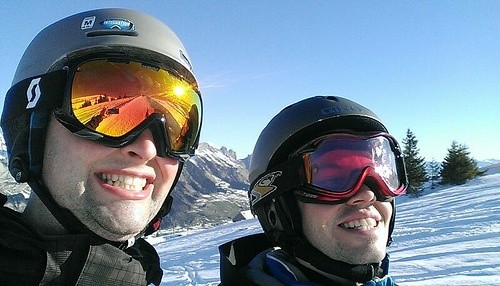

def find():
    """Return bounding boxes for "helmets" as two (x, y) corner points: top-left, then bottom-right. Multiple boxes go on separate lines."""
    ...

(247, 94), (397, 255)
(2, 7), (201, 111)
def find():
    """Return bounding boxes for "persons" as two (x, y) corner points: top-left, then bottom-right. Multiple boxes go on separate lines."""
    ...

(0, 8), (203, 286)
(216, 96), (407, 286)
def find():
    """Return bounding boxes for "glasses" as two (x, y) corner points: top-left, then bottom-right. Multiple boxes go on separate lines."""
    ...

(248, 130), (409, 200)
(0, 53), (205, 162)
